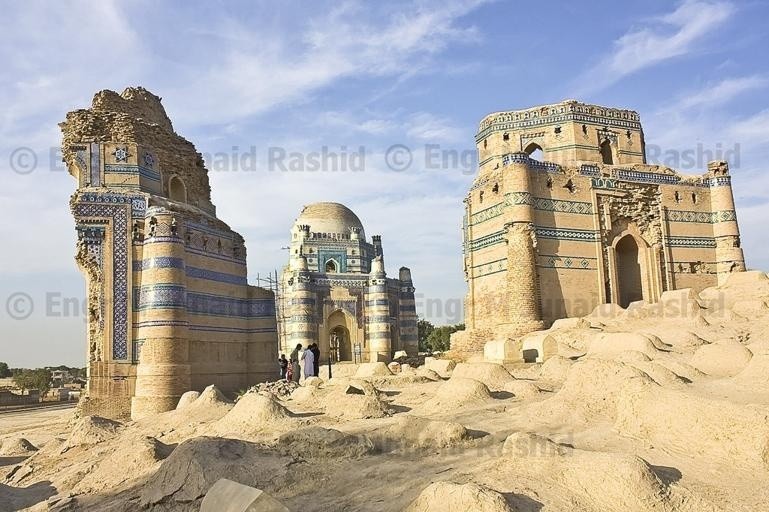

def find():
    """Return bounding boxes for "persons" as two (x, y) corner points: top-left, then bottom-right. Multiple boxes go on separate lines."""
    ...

(276, 342), (319, 382)
